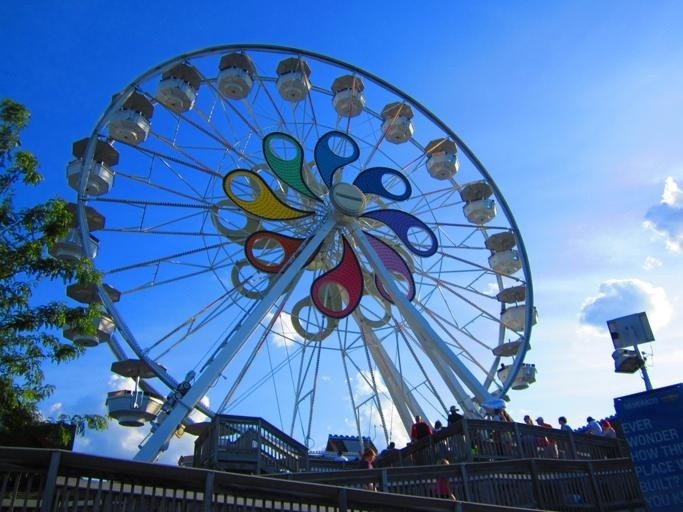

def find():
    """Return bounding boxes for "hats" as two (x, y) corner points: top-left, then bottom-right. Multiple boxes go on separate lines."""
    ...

(450, 406), (458, 410)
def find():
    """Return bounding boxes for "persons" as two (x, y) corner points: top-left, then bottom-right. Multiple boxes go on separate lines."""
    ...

(376, 404), (622, 466)
(431, 456), (458, 500)
(355, 446), (377, 492)
(334, 450), (347, 470)
